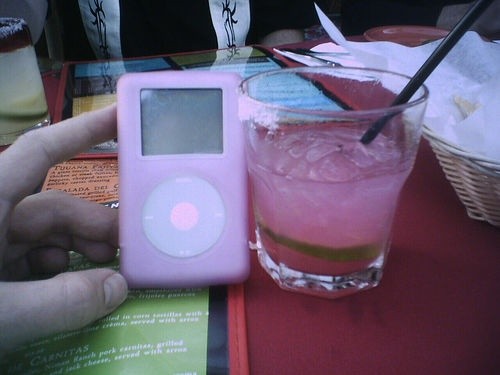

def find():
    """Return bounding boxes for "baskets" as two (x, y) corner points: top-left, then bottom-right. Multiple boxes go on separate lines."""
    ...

(420, 127), (500, 226)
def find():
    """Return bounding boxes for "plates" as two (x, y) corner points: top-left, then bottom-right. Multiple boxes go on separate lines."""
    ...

(363, 25), (451, 46)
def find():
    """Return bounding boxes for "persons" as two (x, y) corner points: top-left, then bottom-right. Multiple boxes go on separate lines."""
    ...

(61, 0), (306, 63)
(0, 101), (128, 353)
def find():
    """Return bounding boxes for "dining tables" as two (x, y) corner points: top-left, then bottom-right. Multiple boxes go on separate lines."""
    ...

(0, 35), (500, 375)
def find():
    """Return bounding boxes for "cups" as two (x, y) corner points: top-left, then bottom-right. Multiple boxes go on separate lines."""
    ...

(237, 66), (430, 299)
(0, 17), (51, 146)
